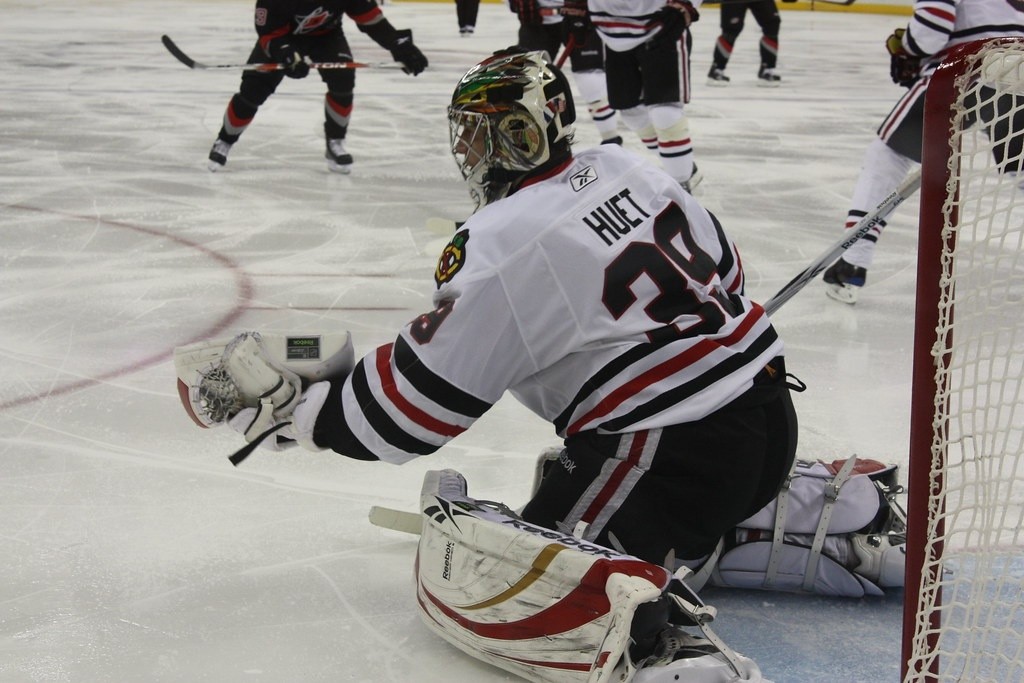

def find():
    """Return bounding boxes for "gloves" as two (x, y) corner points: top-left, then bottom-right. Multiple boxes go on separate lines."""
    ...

(887, 29), (921, 89)
(509, 0), (543, 34)
(644, 0), (699, 53)
(275, 44), (309, 79)
(557, 0), (594, 52)
(390, 29), (429, 77)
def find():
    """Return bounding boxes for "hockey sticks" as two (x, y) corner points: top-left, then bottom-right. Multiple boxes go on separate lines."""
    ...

(161, 34), (407, 73)
(366, 164), (924, 538)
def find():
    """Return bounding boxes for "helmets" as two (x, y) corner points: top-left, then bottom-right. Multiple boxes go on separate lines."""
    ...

(446, 48), (576, 206)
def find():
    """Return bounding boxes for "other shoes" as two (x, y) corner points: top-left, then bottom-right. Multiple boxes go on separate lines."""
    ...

(459, 25), (474, 37)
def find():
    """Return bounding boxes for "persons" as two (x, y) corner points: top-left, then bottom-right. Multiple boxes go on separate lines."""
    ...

(508, 0), (703, 193)
(175, 49), (907, 683)
(210, 0), (430, 173)
(707, 0), (781, 82)
(454, 1), (480, 36)
(823, 1), (1024, 307)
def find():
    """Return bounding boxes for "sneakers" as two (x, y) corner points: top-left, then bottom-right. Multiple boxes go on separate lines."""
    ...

(823, 259), (867, 303)
(323, 138), (353, 173)
(707, 66), (729, 85)
(208, 135), (233, 171)
(757, 65), (780, 86)
